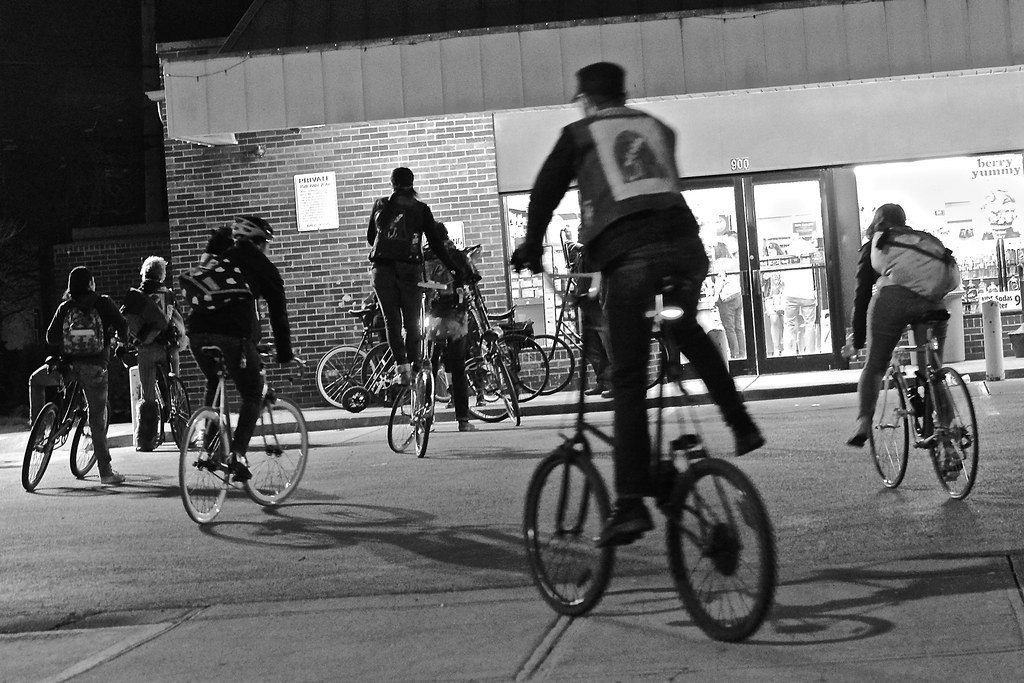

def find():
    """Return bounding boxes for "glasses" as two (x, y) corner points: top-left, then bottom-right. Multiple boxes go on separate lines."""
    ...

(265, 239), (271, 245)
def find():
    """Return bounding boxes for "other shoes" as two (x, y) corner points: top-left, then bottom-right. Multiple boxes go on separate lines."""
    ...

(135, 447), (152, 453)
(32, 435), (44, 449)
(731, 419), (766, 456)
(847, 417), (873, 447)
(600, 389), (614, 397)
(596, 498), (654, 550)
(101, 470), (126, 483)
(584, 385), (605, 395)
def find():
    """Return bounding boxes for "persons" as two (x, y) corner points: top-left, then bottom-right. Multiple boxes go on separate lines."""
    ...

(509, 62), (765, 545)
(840, 204), (963, 474)
(566, 224), (614, 397)
(711, 238), (820, 359)
(114, 255), (196, 451)
(367, 167), (473, 385)
(28, 267), (128, 484)
(420, 222), (479, 432)
(184, 215), (294, 479)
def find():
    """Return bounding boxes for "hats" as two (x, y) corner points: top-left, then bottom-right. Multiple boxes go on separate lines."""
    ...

(872, 204), (905, 227)
(572, 61), (627, 103)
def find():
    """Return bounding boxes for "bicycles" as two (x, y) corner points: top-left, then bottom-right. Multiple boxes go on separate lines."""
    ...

(868, 309), (982, 500)
(313, 239), (672, 461)
(522, 247), (784, 641)
(179, 344), (308, 526)
(20, 339), (120, 493)
(116, 342), (192, 451)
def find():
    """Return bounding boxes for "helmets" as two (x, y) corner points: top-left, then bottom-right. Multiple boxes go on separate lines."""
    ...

(232, 214), (276, 241)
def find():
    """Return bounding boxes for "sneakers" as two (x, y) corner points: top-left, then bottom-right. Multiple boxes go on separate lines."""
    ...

(444, 399), (454, 409)
(228, 452), (254, 480)
(459, 422), (473, 430)
(476, 395), (486, 406)
(393, 364), (411, 384)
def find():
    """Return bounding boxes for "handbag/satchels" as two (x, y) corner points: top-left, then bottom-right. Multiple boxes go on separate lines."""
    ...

(428, 294), (468, 341)
(178, 250), (257, 316)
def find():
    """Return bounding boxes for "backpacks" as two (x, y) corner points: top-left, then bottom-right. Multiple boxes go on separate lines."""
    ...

(868, 224), (959, 302)
(60, 291), (105, 358)
(125, 284), (169, 343)
(370, 199), (421, 263)
